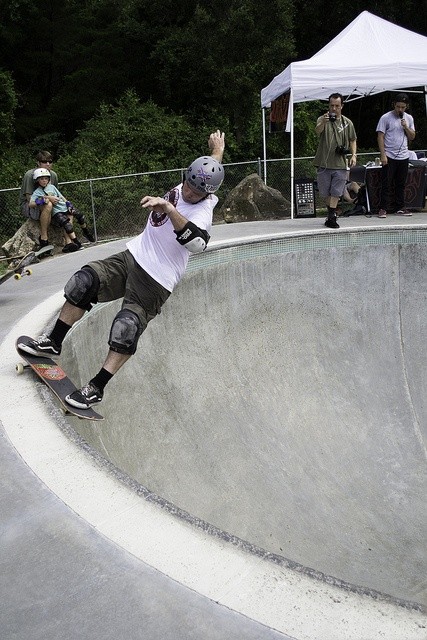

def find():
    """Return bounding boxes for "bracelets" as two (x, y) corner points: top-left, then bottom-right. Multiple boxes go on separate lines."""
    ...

(352, 153), (358, 158)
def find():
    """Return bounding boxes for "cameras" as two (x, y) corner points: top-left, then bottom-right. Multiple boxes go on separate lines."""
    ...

(328, 113), (336, 122)
(335, 145), (345, 155)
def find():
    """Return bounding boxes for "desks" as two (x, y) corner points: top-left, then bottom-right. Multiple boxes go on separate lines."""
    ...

(351, 159), (427, 215)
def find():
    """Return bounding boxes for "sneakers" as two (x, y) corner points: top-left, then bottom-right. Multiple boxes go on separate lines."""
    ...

(395, 209), (412, 216)
(325, 217), (340, 229)
(65, 382), (103, 410)
(82, 232), (95, 242)
(62, 243), (79, 253)
(19, 333), (62, 360)
(38, 235), (50, 255)
(73, 238), (84, 248)
(378, 209), (386, 218)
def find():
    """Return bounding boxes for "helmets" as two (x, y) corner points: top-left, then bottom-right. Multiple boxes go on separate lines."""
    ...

(33, 168), (51, 184)
(184, 156), (225, 198)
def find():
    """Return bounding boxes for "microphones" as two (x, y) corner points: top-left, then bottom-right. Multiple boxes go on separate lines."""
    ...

(398, 113), (405, 130)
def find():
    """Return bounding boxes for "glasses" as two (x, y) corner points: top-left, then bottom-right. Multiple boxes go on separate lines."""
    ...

(40, 159), (53, 163)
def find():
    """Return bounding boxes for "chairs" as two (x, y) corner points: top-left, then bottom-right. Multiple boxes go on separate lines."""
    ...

(339, 165), (371, 218)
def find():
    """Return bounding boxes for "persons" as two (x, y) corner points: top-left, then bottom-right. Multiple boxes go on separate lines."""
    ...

(28, 168), (96, 250)
(17, 129), (227, 408)
(19, 149), (80, 254)
(375, 92), (416, 220)
(311, 92), (358, 227)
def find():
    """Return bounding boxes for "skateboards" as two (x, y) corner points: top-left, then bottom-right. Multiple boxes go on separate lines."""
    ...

(0, 251), (35, 285)
(15, 335), (106, 421)
(35, 245), (54, 263)
